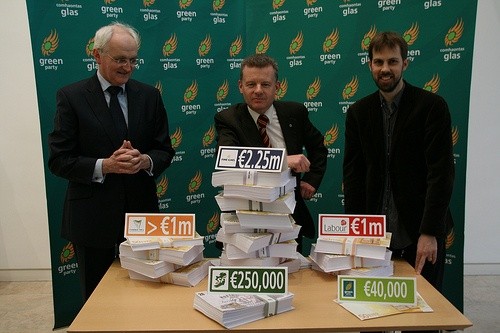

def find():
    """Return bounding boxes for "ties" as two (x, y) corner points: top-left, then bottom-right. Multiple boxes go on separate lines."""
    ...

(257, 115), (271, 149)
(106, 87), (127, 136)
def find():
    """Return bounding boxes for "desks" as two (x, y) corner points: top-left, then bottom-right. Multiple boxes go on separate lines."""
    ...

(67, 257), (473, 333)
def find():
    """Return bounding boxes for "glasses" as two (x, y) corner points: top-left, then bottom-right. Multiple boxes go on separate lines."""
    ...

(100, 52), (139, 66)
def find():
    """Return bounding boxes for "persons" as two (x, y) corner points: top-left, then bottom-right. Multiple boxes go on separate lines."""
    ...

(213, 53), (328, 256)
(343, 32), (454, 290)
(46, 22), (175, 304)
